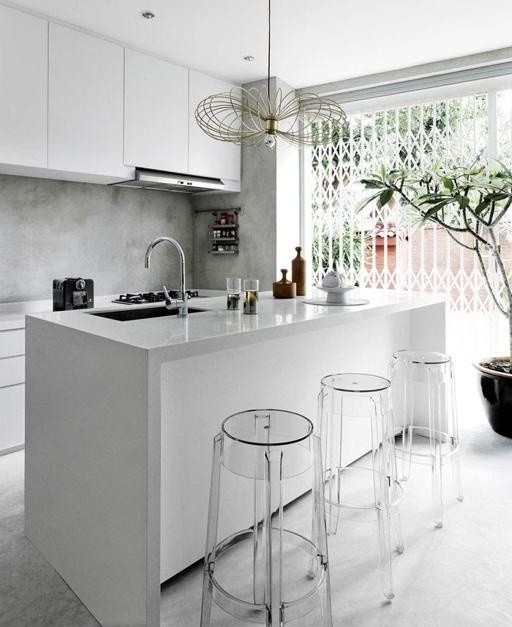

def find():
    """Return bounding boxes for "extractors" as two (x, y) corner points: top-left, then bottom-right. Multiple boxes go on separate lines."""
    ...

(110, 169), (225, 194)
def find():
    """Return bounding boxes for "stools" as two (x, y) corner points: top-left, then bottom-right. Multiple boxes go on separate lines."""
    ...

(385, 350), (462, 532)
(199, 407), (330, 622)
(307, 373), (402, 604)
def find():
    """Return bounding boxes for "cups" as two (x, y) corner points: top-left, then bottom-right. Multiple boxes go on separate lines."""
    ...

(243, 279), (259, 315)
(225, 278), (241, 311)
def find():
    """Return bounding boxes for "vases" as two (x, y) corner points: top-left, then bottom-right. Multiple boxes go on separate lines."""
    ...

(272, 268), (297, 298)
(292, 245), (307, 296)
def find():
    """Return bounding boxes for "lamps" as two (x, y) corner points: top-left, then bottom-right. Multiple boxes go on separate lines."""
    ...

(194, 0), (350, 151)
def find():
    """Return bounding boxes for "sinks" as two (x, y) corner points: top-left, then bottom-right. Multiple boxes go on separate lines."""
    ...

(86, 304), (210, 323)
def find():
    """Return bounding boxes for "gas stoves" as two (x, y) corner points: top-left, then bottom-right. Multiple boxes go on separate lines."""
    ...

(115, 290), (200, 305)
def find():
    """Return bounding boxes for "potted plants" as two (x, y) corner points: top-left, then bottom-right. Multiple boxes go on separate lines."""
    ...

(354, 159), (512, 441)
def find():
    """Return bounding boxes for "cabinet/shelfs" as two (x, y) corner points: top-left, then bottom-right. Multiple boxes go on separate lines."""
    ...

(210, 222), (239, 254)
(1, 327), (26, 452)
(0, 5), (136, 185)
(122, 46), (242, 196)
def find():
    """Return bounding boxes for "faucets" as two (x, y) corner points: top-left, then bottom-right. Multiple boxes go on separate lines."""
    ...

(144, 235), (190, 319)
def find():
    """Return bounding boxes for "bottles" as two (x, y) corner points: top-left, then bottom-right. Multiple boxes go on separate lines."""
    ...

(212, 228), (237, 239)
(212, 241), (238, 252)
(212, 212), (235, 225)
